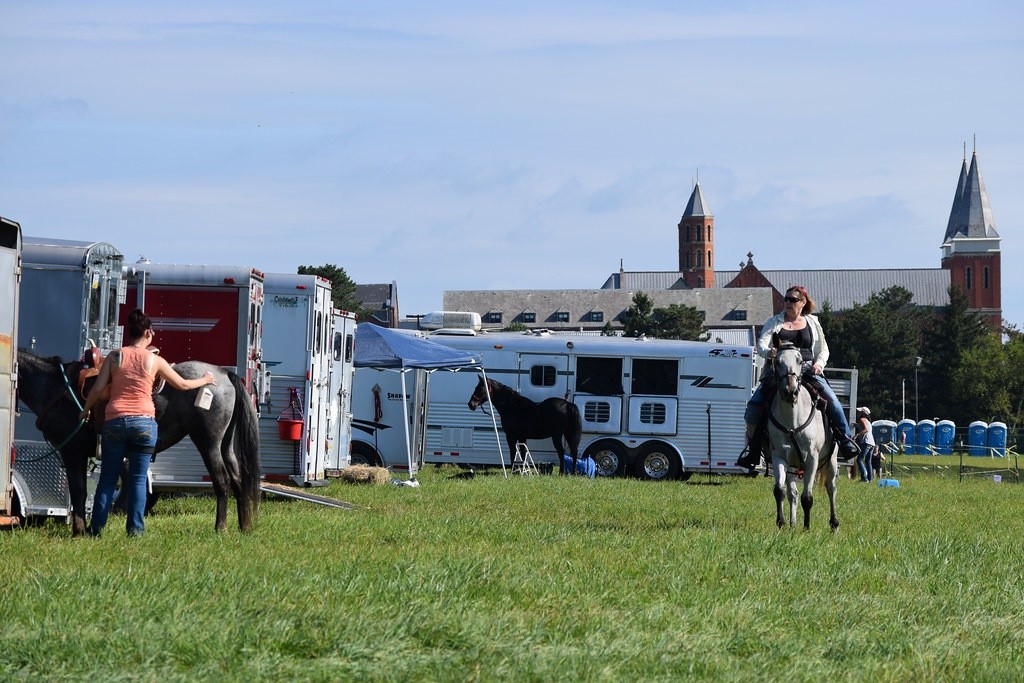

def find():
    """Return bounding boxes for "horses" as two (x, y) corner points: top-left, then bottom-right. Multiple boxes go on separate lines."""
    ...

(468, 374), (583, 477)
(756, 332), (840, 531)
(16, 346), (262, 538)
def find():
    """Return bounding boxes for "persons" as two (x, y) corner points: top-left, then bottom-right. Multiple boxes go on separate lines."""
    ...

(737, 286), (859, 470)
(846, 406), (886, 484)
(78, 309), (218, 538)
(900, 430), (907, 444)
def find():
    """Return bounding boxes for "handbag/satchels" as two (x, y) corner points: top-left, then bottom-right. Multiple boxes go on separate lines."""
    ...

(878, 451), (886, 463)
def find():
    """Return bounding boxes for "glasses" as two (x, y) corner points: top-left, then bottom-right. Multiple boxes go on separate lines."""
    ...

(144, 328), (155, 336)
(783, 296), (804, 303)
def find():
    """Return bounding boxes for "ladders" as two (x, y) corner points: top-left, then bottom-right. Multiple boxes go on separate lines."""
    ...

(511, 443), (540, 476)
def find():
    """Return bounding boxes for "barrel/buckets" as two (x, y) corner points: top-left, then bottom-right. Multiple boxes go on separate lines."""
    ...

(277, 404), (304, 440)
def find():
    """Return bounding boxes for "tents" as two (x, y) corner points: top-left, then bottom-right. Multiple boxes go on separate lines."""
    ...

(351, 321), (509, 482)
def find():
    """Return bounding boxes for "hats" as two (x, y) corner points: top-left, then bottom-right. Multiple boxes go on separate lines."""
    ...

(856, 406), (870, 415)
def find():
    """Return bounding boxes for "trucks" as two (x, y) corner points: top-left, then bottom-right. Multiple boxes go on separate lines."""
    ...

(1, 217), (361, 533)
(352, 328), (761, 482)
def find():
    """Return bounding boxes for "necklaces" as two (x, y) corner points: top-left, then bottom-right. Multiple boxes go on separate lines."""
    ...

(790, 325), (792, 329)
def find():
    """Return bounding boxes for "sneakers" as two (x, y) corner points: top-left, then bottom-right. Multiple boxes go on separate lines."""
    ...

(738, 453), (760, 466)
(859, 477), (869, 483)
(840, 434), (858, 459)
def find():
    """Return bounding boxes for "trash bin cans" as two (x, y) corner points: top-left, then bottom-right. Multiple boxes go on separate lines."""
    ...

(897, 418), (916, 455)
(917, 419), (937, 455)
(968, 421), (989, 457)
(871, 420), (897, 454)
(936, 420), (956, 455)
(987, 422), (1007, 457)
